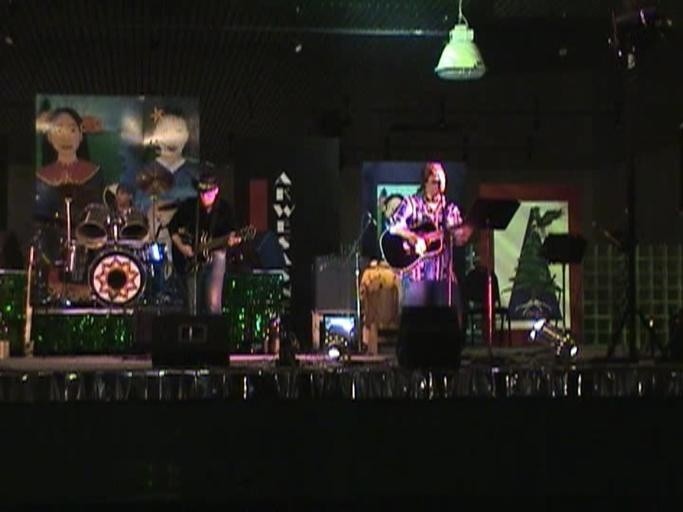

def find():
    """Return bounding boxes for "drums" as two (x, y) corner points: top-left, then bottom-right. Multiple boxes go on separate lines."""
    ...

(117, 207), (149, 239)
(86, 247), (147, 305)
(62, 239), (94, 274)
(74, 202), (113, 250)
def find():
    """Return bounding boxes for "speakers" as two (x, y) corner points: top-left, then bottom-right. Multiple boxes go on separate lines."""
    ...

(397, 306), (461, 371)
(152, 315), (229, 369)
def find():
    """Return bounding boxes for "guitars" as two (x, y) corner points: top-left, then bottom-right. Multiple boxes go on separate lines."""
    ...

(380, 209), (492, 272)
(172, 224), (257, 276)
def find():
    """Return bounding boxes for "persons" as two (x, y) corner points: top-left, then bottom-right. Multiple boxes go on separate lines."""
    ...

(35, 108), (200, 209)
(168, 174), (242, 315)
(388, 160), (474, 382)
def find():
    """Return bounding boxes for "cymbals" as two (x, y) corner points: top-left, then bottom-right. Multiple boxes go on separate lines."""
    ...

(137, 166), (173, 194)
(33, 214), (73, 231)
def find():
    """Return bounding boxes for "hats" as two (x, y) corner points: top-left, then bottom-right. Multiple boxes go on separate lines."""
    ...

(192, 174), (219, 192)
(422, 162), (448, 185)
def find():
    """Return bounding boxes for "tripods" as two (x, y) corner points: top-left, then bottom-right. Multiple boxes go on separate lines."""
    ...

(603, 55), (670, 363)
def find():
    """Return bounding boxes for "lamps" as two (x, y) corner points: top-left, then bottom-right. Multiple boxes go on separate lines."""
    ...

(434, 2), (487, 82)
